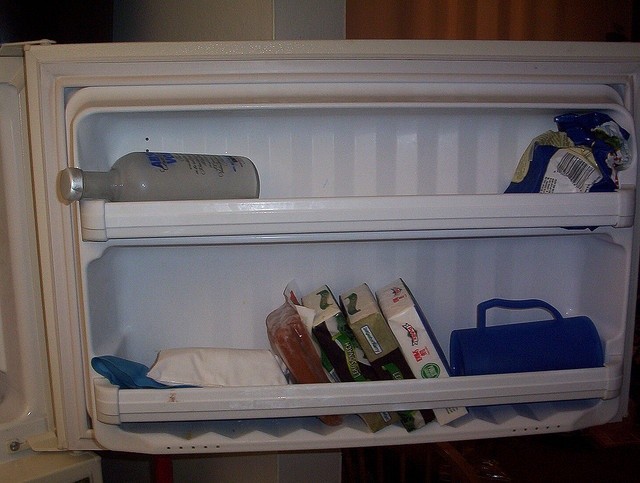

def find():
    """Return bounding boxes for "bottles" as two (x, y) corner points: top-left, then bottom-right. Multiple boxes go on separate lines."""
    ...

(60, 152), (260, 202)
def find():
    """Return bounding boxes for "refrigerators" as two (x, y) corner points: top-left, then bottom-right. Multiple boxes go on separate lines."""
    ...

(0, 41), (639, 482)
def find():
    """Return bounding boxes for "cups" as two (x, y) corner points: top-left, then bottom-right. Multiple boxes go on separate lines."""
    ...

(450, 300), (603, 378)
